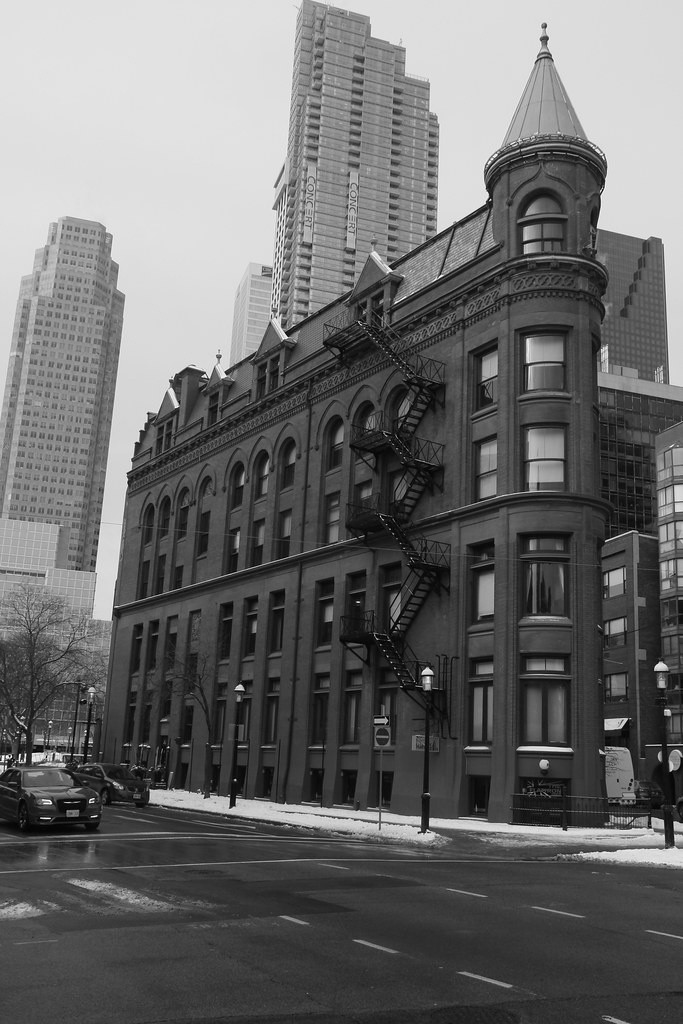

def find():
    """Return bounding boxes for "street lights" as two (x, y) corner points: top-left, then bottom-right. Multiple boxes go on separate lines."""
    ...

(654, 657), (673, 846)
(421, 664), (434, 835)
(82, 686), (97, 765)
(228, 680), (247, 808)
(65, 727), (73, 754)
(46, 719), (54, 750)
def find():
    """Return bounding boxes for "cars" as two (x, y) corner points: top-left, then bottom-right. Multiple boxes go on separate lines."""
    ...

(73, 762), (150, 809)
(0, 764), (104, 834)
